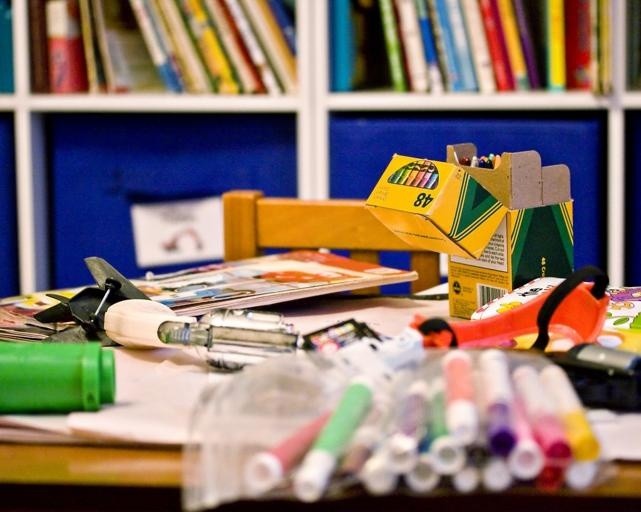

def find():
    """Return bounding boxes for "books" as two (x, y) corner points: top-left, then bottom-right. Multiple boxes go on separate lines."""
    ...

(30, 0), (294, 96)
(333, 0), (616, 94)
(1, 246), (419, 342)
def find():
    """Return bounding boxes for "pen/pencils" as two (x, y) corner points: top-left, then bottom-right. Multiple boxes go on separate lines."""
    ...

(388, 160), (439, 189)
(459, 153), (501, 170)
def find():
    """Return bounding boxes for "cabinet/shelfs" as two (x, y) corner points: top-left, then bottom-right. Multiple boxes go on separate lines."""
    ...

(1, 1), (641, 285)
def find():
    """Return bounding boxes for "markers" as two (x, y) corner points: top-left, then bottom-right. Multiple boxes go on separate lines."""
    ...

(0, 342), (117, 414)
(246, 347), (601, 504)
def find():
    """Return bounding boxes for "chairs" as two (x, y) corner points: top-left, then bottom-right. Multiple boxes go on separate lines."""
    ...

(223, 190), (439, 296)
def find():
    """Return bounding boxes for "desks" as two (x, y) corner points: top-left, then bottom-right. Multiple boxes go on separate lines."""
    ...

(1, 298), (640, 511)
